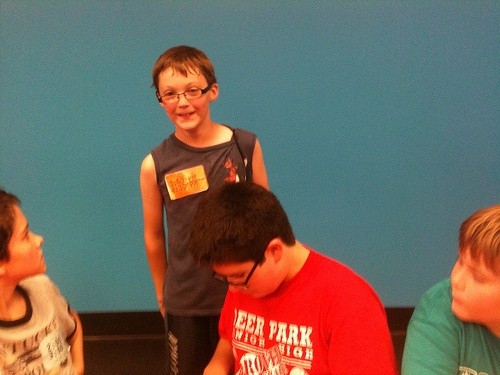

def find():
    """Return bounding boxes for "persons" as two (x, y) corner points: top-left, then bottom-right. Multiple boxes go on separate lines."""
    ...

(140, 45), (270, 375)
(190, 180), (400, 375)
(400, 204), (500, 375)
(0, 187), (84, 375)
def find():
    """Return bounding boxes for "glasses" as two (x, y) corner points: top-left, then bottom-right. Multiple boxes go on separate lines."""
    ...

(212, 261), (258, 286)
(159, 84), (212, 104)
